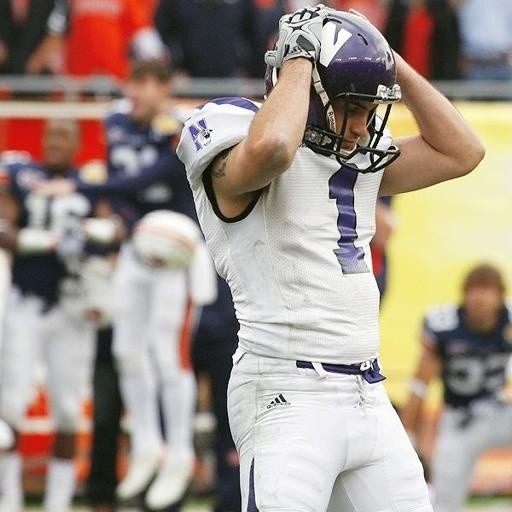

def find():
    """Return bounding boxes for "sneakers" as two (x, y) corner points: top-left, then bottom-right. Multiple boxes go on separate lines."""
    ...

(117, 443), (196, 508)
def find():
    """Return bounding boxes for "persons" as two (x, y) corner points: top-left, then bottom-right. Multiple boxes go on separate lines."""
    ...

(403, 266), (512, 512)
(0, 0), (511, 102)
(0, 62), (240, 511)
(177, 4), (486, 512)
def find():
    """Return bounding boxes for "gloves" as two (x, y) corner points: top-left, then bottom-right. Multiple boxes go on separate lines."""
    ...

(264, 3), (329, 65)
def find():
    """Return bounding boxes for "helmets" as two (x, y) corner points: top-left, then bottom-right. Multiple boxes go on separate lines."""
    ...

(266, 12), (397, 152)
